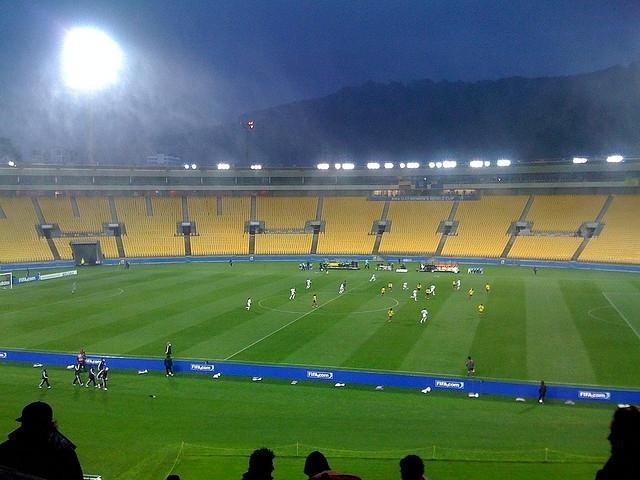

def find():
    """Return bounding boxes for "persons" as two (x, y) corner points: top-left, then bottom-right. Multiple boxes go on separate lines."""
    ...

(302, 450), (361, 480)
(594, 404), (640, 480)
(287, 256), (491, 325)
(123, 257), (129, 269)
(24, 266), (30, 278)
(240, 446), (276, 479)
(244, 297), (252, 312)
(163, 341), (176, 377)
(389, 189), (474, 196)
(38, 363), (52, 389)
(0, 400), (84, 480)
(71, 278), (81, 294)
(535, 381), (546, 406)
(228, 258), (233, 267)
(464, 356), (475, 376)
(116, 256), (126, 271)
(72, 349), (109, 391)
(533, 266), (539, 275)
(399, 455), (426, 480)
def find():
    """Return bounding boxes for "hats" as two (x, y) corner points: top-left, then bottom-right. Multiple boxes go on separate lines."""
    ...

(304, 451), (331, 473)
(15, 401), (53, 425)
